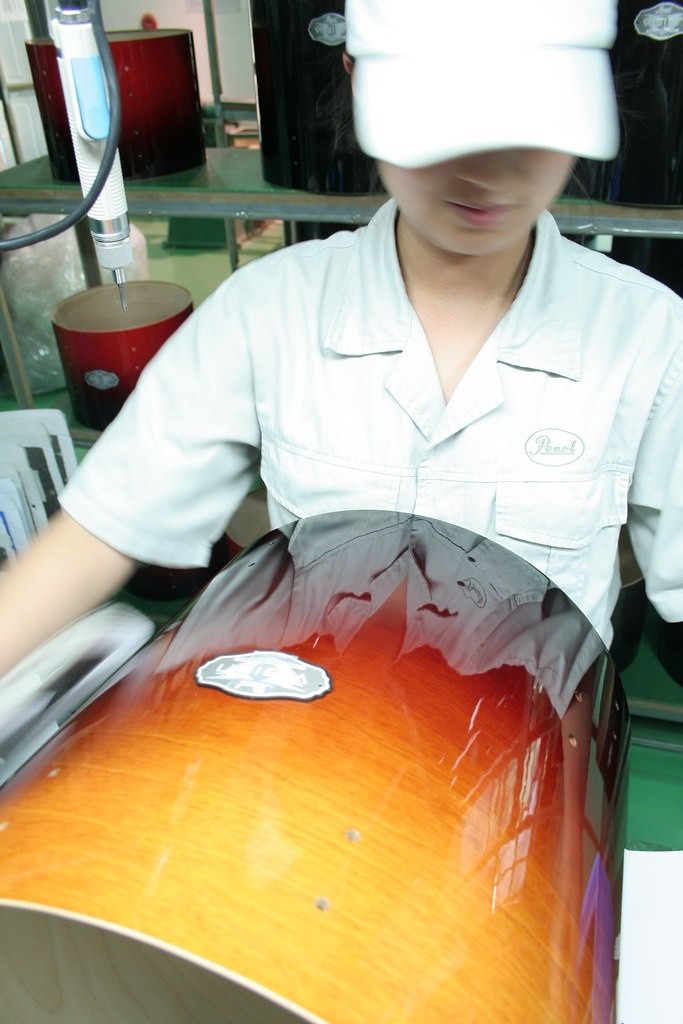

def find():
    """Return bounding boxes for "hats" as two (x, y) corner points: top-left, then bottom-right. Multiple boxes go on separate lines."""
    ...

(344, 0), (622, 168)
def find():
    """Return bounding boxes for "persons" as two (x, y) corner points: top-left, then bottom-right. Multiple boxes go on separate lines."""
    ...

(0, 0), (683, 676)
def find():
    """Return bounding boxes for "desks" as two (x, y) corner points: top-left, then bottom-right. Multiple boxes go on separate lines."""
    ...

(0, 147), (683, 447)
